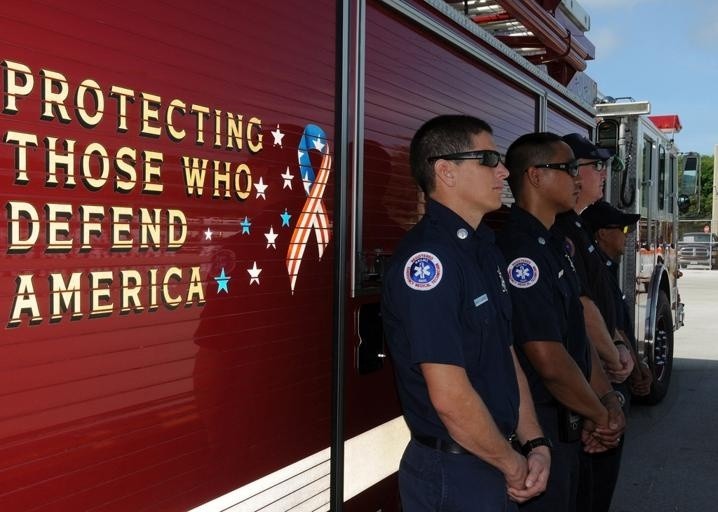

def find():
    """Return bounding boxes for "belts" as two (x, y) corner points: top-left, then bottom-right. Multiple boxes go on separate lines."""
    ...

(431, 433), (518, 456)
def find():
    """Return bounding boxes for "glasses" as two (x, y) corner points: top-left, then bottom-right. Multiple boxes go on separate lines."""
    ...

(578, 160), (607, 172)
(524, 160), (581, 177)
(605, 225), (629, 234)
(428, 149), (505, 169)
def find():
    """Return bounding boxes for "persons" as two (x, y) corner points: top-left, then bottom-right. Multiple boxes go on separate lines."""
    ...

(378, 110), (558, 511)
(549, 129), (636, 511)
(577, 195), (653, 511)
(495, 133), (627, 511)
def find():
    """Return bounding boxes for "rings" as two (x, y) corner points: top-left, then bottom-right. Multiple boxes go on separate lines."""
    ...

(616, 437), (620, 443)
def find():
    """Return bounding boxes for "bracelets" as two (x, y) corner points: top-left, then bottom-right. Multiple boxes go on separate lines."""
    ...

(599, 389), (626, 412)
(518, 437), (553, 454)
(612, 338), (630, 352)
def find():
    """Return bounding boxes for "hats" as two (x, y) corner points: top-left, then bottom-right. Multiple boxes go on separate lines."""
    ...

(562, 133), (612, 160)
(582, 200), (640, 233)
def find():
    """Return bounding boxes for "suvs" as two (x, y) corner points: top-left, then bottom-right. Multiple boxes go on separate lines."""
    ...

(676, 231), (717, 269)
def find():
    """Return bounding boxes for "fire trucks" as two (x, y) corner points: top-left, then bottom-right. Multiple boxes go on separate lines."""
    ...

(0, 1), (701, 510)
